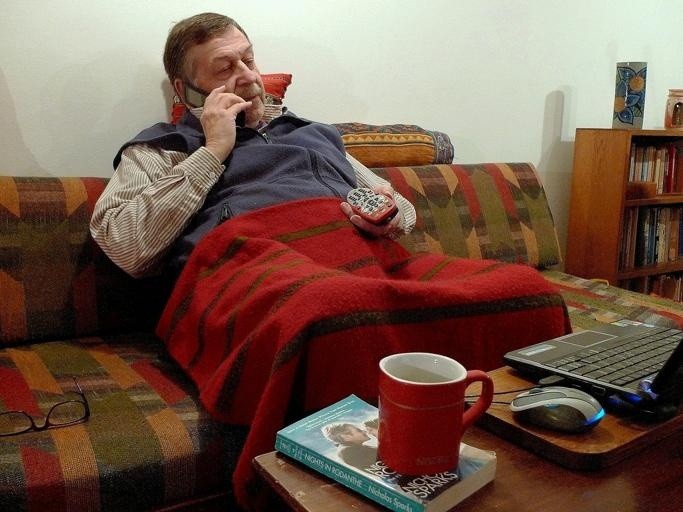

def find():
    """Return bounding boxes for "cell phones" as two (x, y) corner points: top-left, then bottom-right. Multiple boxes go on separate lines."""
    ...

(184, 81), (246, 128)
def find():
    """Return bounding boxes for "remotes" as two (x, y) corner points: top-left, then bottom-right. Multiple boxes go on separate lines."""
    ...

(347, 187), (399, 226)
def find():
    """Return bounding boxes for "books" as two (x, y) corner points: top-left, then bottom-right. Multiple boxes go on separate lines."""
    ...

(615, 135), (683, 300)
(274, 392), (495, 512)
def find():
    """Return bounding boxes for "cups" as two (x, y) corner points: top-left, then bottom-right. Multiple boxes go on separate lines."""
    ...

(373, 351), (493, 477)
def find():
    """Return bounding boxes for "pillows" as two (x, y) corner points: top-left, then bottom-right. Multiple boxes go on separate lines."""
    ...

(172, 74), (292, 125)
(332, 123), (454, 168)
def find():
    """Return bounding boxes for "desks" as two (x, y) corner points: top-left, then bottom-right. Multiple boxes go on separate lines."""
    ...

(251, 425), (683, 512)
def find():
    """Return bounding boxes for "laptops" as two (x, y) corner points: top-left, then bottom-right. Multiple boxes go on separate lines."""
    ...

(504, 319), (683, 424)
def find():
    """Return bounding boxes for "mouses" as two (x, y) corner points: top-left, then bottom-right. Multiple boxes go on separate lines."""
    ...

(510, 386), (605, 433)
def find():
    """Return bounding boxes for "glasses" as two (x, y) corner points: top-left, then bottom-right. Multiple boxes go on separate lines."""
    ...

(0, 374), (90, 435)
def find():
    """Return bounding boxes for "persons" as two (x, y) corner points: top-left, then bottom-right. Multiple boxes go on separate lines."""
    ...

(362, 416), (380, 438)
(319, 421), (380, 470)
(85, 10), (573, 421)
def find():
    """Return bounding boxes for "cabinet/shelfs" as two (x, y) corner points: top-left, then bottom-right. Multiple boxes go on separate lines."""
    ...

(565, 128), (683, 302)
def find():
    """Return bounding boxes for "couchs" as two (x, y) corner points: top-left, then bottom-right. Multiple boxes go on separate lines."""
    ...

(0, 162), (683, 512)
